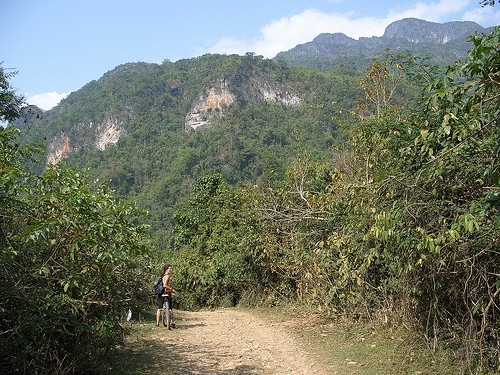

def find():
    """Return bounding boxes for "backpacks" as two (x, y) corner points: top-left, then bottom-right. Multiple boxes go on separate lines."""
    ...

(153, 274), (166, 295)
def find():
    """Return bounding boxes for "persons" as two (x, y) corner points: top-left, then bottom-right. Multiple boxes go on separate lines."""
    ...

(156, 263), (178, 328)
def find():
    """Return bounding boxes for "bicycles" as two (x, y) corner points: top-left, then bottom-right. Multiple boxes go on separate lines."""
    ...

(160, 287), (176, 330)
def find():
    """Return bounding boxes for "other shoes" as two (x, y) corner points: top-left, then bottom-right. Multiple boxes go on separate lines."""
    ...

(171, 324), (176, 328)
(155, 324), (159, 326)
